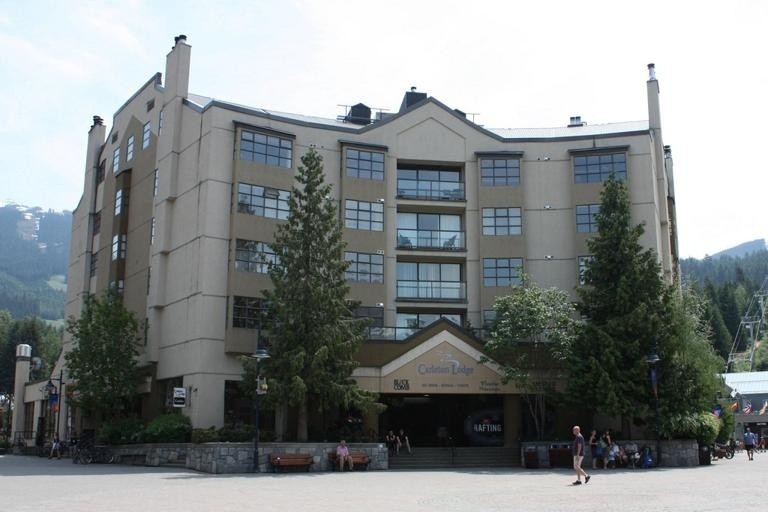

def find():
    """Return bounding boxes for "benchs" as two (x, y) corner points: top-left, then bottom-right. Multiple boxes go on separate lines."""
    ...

(328, 453), (371, 470)
(271, 453), (313, 472)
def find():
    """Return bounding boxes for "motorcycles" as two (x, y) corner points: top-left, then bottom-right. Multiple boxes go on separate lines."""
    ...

(712, 443), (733, 459)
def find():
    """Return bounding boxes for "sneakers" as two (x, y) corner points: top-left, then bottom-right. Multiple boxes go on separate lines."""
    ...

(572, 475), (590, 485)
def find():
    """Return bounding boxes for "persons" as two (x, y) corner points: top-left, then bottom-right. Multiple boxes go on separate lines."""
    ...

(385, 430), (396, 457)
(47, 429), (61, 460)
(337, 440), (354, 472)
(743, 428), (765, 460)
(572, 426), (640, 485)
(396, 429), (412, 457)
(69, 429), (78, 458)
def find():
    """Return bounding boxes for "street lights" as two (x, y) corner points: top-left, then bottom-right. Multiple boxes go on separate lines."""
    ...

(253, 315), (272, 474)
(51, 368), (63, 459)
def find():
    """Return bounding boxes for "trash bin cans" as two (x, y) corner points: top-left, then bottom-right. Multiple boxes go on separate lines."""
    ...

(549, 444), (574, 467)
(699, 444), (710, 465)
(524, 445), (539, 468)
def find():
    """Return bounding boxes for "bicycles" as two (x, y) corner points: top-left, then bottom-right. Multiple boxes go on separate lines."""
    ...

(71, 437), (115, 465)
